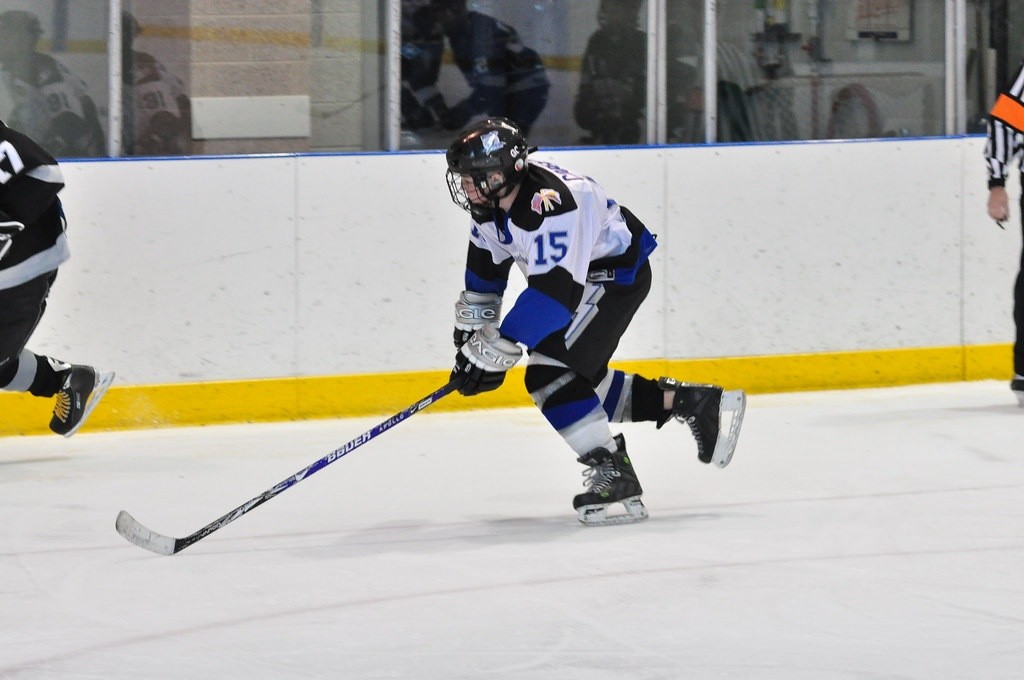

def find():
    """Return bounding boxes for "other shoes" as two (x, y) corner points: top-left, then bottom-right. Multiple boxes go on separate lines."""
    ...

(1011, 373), (1024, 405)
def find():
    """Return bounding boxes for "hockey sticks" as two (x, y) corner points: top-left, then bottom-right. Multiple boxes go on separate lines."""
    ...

(112, 381), (462, 558)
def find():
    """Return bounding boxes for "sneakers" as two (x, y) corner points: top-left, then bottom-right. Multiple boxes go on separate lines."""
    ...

(652, 376), (745, 468)
(571, 433), (650, 524)
(49, 365), (115, 438)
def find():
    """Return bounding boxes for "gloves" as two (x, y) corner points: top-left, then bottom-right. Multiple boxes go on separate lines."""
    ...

(453, 324), (522, 395)
(452, 292), (502, 353)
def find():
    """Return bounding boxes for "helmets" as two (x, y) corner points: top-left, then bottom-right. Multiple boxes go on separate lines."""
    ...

(446, 116), (538, 180)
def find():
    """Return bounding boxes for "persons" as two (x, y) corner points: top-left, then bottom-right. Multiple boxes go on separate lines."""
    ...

(0, 125), (116, 436)
(0, 10), (193, 157)
(401, 1), (770, 152)
(445, 116), (750, 525)
(987, 66), (1024, 406)
